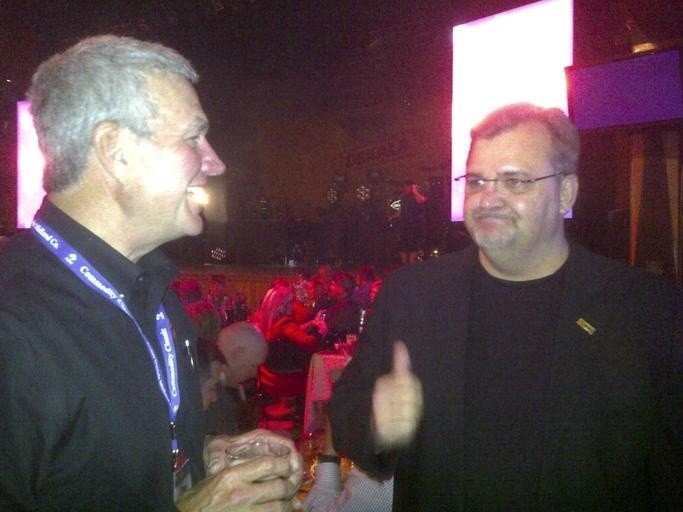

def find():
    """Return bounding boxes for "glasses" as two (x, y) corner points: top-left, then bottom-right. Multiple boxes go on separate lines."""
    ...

(453, 171), (564, 196)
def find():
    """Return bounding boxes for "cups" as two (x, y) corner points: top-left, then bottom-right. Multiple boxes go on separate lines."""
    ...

(225, 441), (292, 488)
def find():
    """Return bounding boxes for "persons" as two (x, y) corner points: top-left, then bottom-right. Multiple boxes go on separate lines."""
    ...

(258, 261), (385, 431)
(184, 178), (424, 266)
(183, 277), (267, 432)
(325, 99), (681, 510)
(0, 32), (306, 512)
